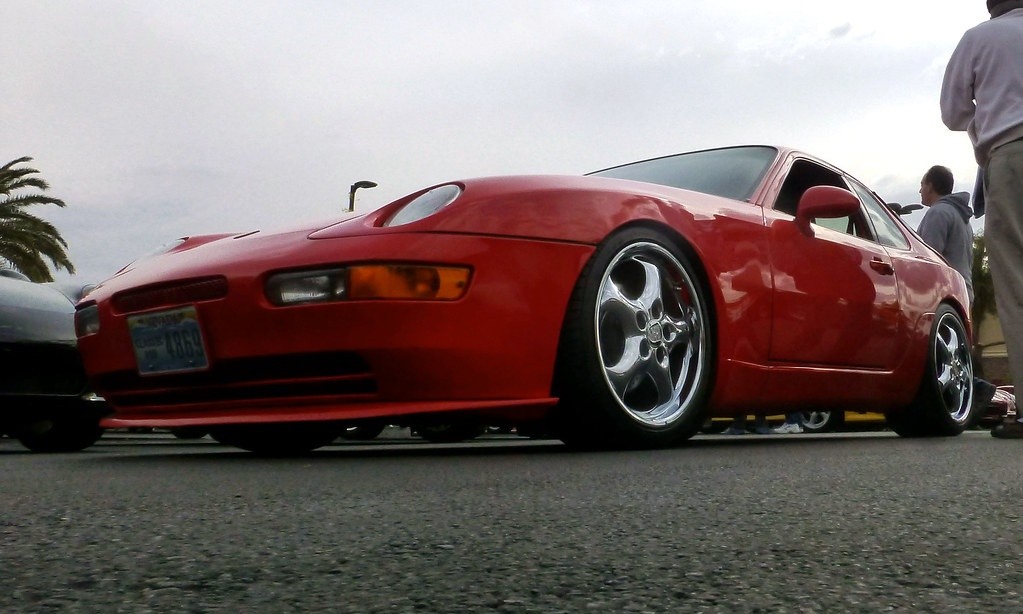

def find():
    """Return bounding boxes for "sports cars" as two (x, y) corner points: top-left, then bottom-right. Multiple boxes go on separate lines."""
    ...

(72, 143), (975, 450)
(0, 251), (105, 454)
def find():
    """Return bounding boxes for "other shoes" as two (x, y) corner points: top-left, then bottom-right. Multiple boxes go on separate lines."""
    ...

(967, 384), (995, 430)
(991, 418), (1023, 439)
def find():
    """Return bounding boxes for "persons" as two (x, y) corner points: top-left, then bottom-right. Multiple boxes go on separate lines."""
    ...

(720, 415), (768, 435)
(940, 0), (1023, 439)
(774, 412), (804, 433)
(916, 165), (974, 303)
